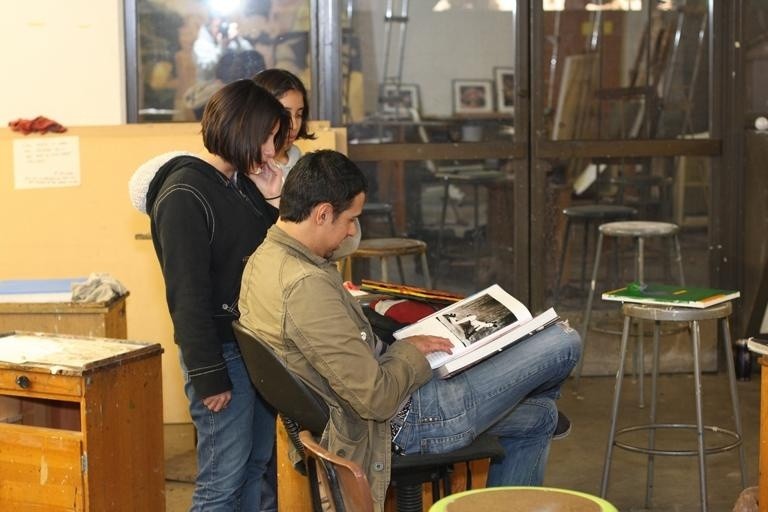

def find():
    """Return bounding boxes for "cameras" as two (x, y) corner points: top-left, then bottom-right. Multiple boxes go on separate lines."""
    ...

(217, 20), (232, 41)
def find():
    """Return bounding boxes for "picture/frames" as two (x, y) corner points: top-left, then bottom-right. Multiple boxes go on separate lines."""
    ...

(377, 83), (421, 115)
(452, 79), (495, 113)
(493, 67), (514, 114)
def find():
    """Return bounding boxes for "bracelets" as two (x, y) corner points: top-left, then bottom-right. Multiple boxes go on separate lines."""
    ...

(263, 194), (281, 201)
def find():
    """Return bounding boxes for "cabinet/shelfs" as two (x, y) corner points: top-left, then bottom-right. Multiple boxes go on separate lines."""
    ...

(1, 291), (131, 341)
(0, 329), (166, 511)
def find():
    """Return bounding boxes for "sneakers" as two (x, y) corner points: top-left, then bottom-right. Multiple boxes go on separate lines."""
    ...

(552, 410), (571, 439)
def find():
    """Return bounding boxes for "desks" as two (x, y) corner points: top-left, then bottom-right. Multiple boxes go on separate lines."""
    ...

(748, 333), (768, 512)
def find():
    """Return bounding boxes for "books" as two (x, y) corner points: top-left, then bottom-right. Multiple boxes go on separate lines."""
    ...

(1, 275), (96, 303)
(552, 53), (601, 141)
(392, 283), (561, 380)
(600, 282), (742, 310)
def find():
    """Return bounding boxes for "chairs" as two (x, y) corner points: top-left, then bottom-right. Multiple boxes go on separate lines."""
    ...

(298, 430), (373, 511)
(231, 319), (505, 512)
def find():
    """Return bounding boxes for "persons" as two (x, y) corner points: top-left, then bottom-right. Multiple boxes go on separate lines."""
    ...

(182, 15), (267, 120)
(243, 68), (317, 208)
(128, 79), (294, 511)
(239, 149), (583, 511)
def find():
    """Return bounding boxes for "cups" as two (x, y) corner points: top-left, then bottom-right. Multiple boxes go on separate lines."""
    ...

(446, 125), (463, 142)
